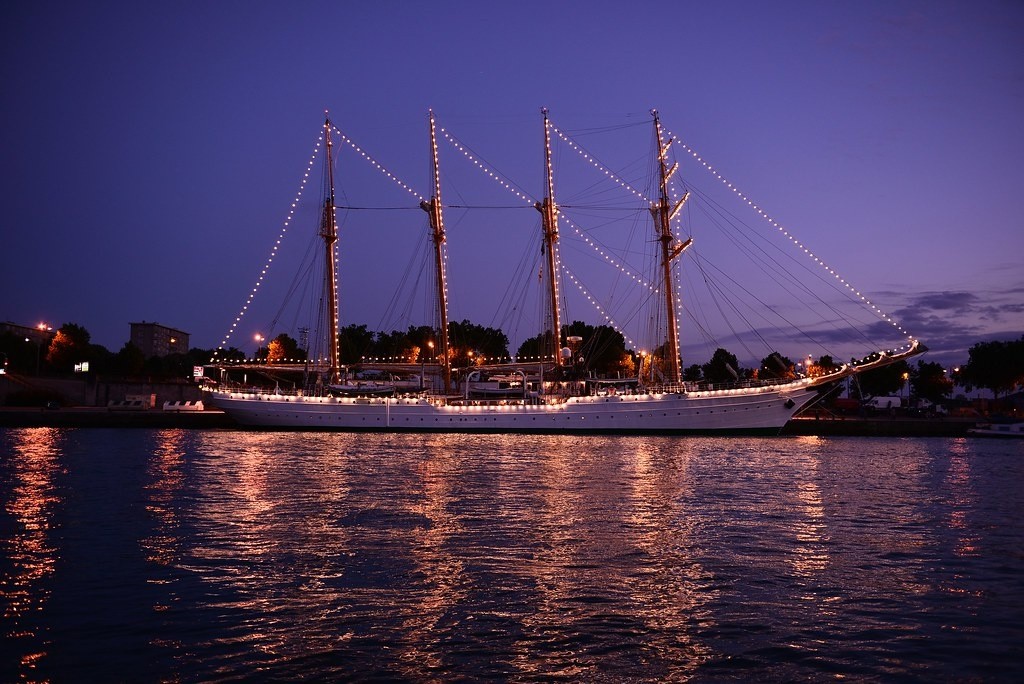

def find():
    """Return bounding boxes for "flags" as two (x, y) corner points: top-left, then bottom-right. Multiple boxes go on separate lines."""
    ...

(538, 263), (542, 283)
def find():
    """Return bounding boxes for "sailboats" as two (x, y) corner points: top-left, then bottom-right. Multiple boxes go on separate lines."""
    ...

(188, 105), (927, 431)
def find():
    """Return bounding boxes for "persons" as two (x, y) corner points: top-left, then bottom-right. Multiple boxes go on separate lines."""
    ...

(608, 387), (615, 396)
(761, 377), (769, 386)
(692, 383), (698, 391)
